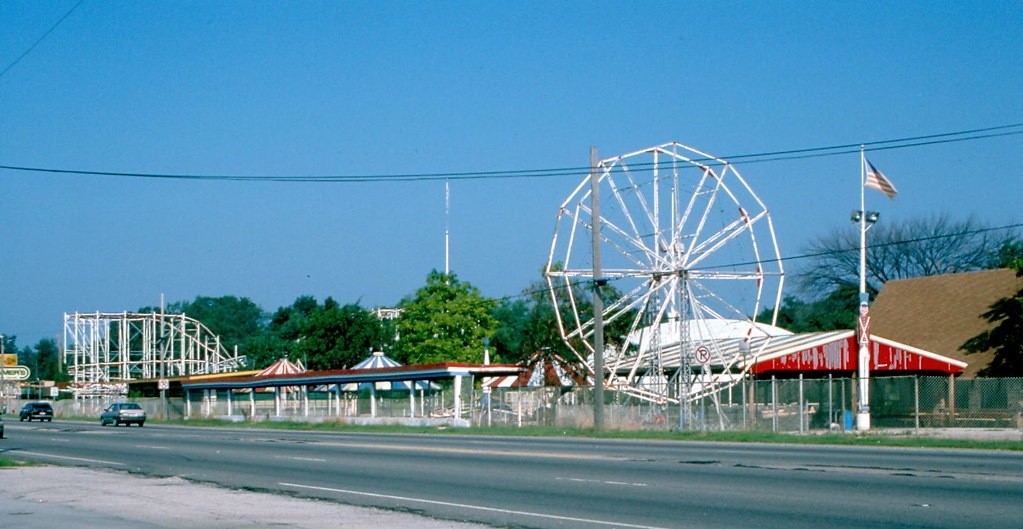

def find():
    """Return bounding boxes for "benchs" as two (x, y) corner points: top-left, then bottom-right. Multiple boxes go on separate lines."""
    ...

(910, 411), (1012, 428)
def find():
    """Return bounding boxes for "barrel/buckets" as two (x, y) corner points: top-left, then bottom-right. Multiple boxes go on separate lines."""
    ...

(844, 410), (852, 431)
(857, 413), (870, 432)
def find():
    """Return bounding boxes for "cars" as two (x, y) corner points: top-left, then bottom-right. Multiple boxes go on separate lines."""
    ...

(0, 411), (6, 440)
(99, 401), (147, 427)
(18, 402), (54, 423)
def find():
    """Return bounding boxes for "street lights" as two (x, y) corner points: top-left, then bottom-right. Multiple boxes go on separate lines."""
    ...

(849, 209), (881, 431)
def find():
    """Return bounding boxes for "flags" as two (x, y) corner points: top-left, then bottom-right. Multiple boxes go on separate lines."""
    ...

(864, 157), (898, 202)
